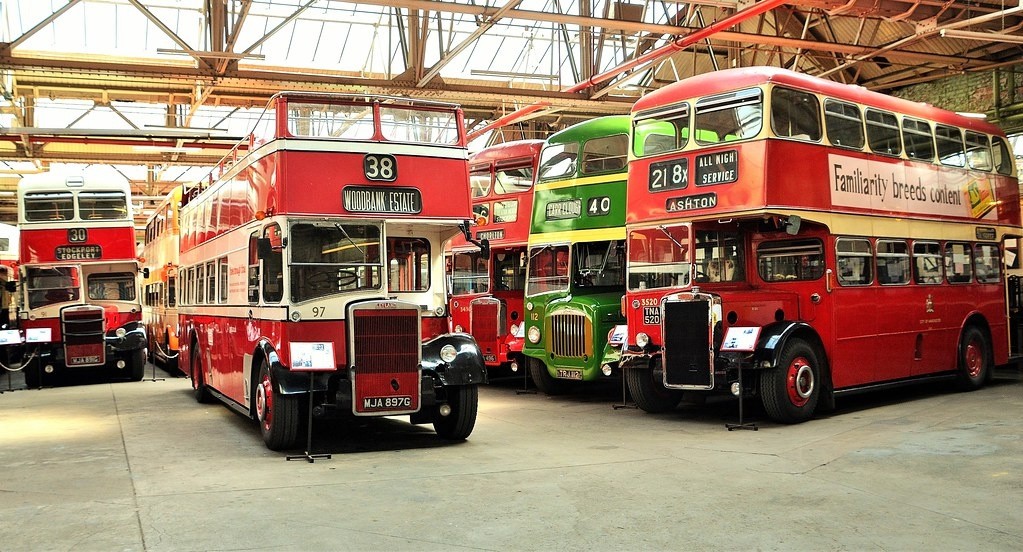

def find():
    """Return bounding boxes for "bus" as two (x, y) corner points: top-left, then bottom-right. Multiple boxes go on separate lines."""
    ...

(137, 184), (211, 380)
(173, 90), (489, 453)
(619, 67), (1022, 426)
(439, 138), (545, 390)
(0, 222), (28, 375)
(16, 164), (152, 389)
(504, 113), (743, 406)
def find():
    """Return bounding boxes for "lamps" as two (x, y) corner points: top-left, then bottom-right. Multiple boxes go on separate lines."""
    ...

(144, 124), (229, 134)
(156, 48), (265, 61)
(471, 69), (559, 81)
(940, 0), (1023, 44)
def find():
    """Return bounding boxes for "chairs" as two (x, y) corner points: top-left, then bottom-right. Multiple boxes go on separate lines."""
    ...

(47, 286), (69, 305)
(881, 262), (905, 283)
